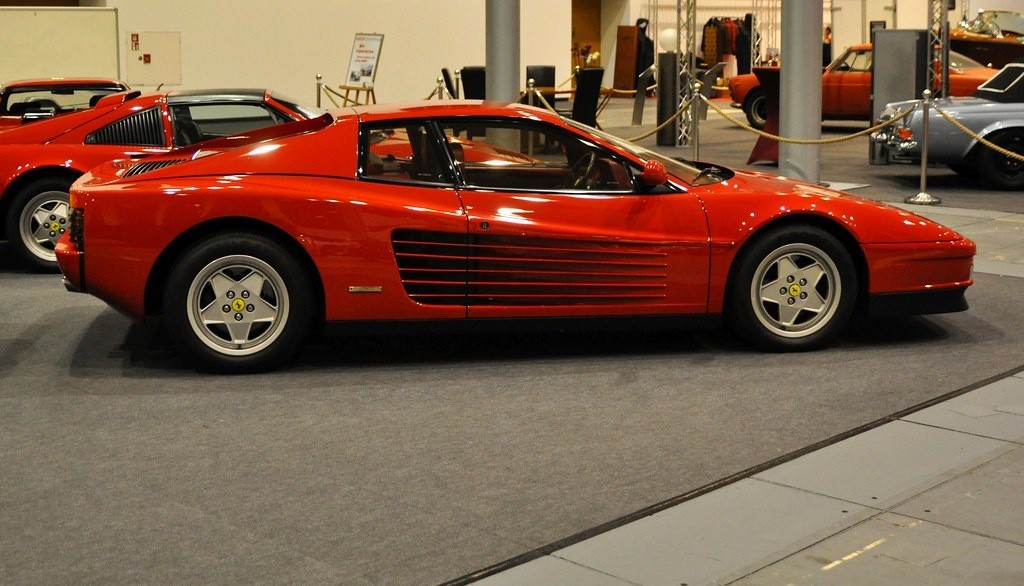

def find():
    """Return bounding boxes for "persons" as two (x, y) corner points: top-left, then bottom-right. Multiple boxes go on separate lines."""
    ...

(730, 14), (760, 107)
(825, 27), (831, 43)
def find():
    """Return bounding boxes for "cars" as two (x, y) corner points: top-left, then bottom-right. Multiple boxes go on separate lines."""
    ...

(870, 63), (1024, 187)
(728, 44), (1002, 130)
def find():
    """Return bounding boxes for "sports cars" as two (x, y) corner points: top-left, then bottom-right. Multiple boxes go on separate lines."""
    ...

(0, 77), (323, 272)
(55, 99), (976, 372)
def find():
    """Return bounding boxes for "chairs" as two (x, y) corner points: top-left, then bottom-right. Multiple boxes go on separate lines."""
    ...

(173, 104), (205, 151)
(443, 63), (605, 129)
(420, 125), (470, 188)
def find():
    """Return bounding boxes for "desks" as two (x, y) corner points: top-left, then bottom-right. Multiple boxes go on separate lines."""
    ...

(519, 83), (614, 131)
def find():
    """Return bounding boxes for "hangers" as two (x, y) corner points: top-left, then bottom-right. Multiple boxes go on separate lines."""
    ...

(704, 14), (747, 31)
